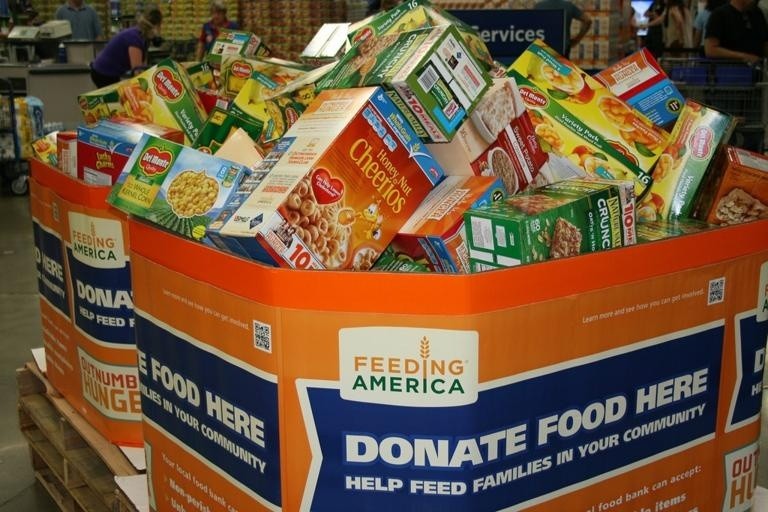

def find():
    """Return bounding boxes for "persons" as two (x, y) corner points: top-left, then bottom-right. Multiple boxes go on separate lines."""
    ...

(5, 1), (40, 32)
(90, 9), (162, 89)
(702, 0), (768, 157)
(364, 0), (406, 17)
(534, 1), (592, 60)
(55, 0), (103, 41)
(629, 0), (712, 71)
(197, 0), (238, 62)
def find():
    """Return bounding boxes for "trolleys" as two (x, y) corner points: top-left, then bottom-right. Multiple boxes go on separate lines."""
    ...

(0, 78), (32, 198)
(657, 57), (768, 148)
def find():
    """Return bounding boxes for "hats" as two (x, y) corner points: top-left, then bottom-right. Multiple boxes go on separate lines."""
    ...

(143, 4), (161, 24)
(211, 2), (226, 12)
